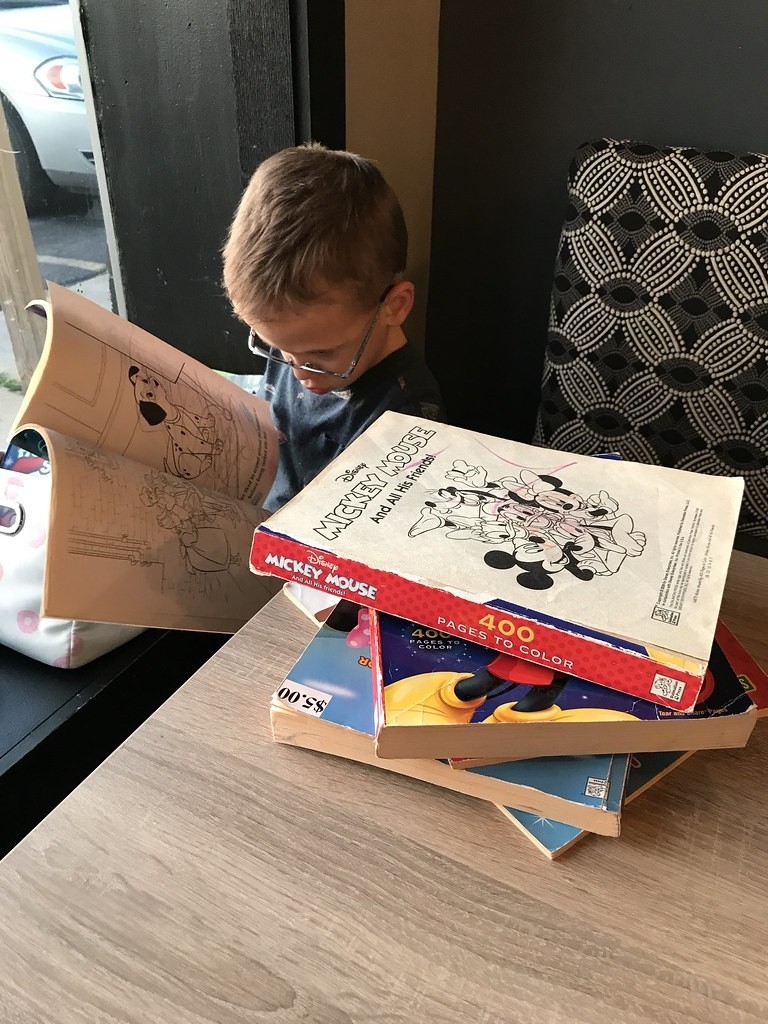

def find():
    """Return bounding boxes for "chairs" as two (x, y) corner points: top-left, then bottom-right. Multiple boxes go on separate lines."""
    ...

(536, 138), (768, 558)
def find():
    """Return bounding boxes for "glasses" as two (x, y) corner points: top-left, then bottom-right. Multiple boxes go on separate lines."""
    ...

(248, 285), (397, 379)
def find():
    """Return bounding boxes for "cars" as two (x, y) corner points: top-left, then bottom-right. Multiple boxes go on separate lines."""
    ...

(0, 0), (100, 217)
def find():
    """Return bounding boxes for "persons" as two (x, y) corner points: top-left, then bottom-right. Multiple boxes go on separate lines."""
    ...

(223, 147), (448, 514)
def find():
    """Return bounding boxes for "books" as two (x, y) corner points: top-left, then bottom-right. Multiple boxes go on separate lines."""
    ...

(447, 615), (768, 771)
(363, 616), (758, 759)
(6, 278), (288, 634)
(283, 581), (342, 628)
(249, 410), (744, 712)
(491, 749), (699, 860)
(269, 598), (632, 838)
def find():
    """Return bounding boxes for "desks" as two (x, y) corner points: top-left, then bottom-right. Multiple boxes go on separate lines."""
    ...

(0, 550), (768, 1024)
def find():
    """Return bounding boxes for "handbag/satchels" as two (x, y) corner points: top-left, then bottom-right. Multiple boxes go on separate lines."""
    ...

(0, 449), (150, 670)
